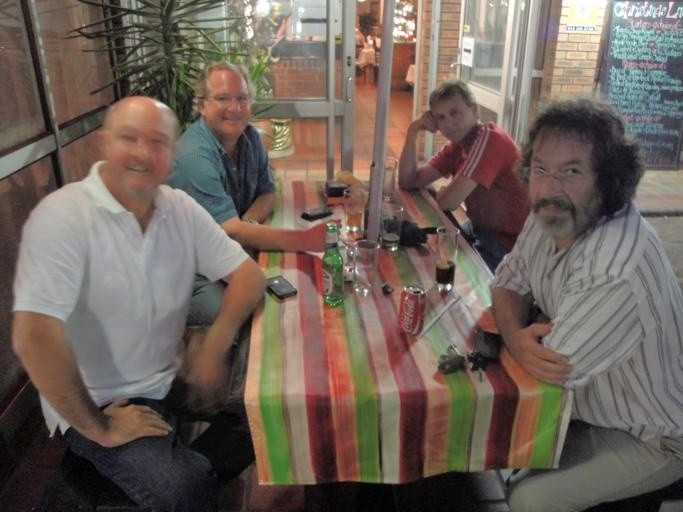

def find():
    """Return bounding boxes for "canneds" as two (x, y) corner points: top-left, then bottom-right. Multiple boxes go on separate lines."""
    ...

(399, 284), (427, 336)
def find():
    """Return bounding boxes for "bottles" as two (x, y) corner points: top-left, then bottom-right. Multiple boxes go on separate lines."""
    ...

(322, 223), (343, 307)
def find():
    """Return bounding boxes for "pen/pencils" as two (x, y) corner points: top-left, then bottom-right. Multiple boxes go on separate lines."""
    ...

(419, 295), (462, 337)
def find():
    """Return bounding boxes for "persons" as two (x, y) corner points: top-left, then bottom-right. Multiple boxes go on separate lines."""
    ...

(397, 77), (532, 271)
(486, 90), (682, 511)
(161, 61), (342, 367)
(10, 94), (267, 511)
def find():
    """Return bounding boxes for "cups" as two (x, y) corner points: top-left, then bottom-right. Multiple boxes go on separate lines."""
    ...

(436, 226), (460, 293)
(382, 157), (398, 203)
(351, 239), (380, 299)
(343, 188), (365, 232)
(380, 199), (406, 253)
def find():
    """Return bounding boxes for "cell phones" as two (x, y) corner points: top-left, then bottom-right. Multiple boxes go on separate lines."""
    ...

(264, 276), (297, 298)
(474, 331), (502, 362)
(301, 206), (332, 220)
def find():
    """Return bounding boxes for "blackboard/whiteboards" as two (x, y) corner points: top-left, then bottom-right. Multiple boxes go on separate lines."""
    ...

(600, 0), (683, 171)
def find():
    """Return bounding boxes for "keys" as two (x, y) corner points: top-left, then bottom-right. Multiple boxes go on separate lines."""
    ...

(473, 359), (485, 382)
